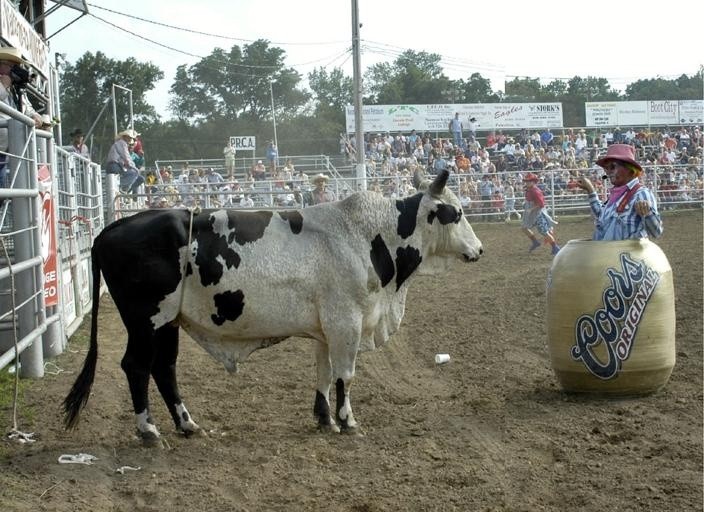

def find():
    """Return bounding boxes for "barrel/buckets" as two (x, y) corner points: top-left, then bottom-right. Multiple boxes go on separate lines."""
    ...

(546, 238), (676, 393)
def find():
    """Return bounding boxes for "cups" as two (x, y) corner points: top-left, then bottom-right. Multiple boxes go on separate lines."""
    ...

(435, 354), (450, 364)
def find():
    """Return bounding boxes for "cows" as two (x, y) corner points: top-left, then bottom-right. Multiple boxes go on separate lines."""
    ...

(57, 165), (485, 452)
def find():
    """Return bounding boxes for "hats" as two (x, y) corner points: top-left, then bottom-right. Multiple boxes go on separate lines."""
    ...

(69, 129), (86, 137)
(522, 175), (539, 182)
(595, 144), (642, 173)
(311, 174), (329, 184)
(470, 118), (477, 121)
(119, 131), (134, 138)
(0, 47), (28, 64)
(508, 138), (515, 144)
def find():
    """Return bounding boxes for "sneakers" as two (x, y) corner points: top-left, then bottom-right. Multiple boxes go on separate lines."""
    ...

(551, 245), (560, 255)
(529, 240), (541, 251)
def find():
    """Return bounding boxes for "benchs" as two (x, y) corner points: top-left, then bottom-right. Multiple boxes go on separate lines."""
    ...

(153, 129), (704, 213)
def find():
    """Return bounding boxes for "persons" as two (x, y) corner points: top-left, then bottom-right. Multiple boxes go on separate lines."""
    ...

(0, 46), (45, 209)
(570, 142), (664, 242)
(106, 130), (337, 207)
(68, 128), (92, 165)
(521, 171), (561, 256)
(339, 111), (704, 221)
